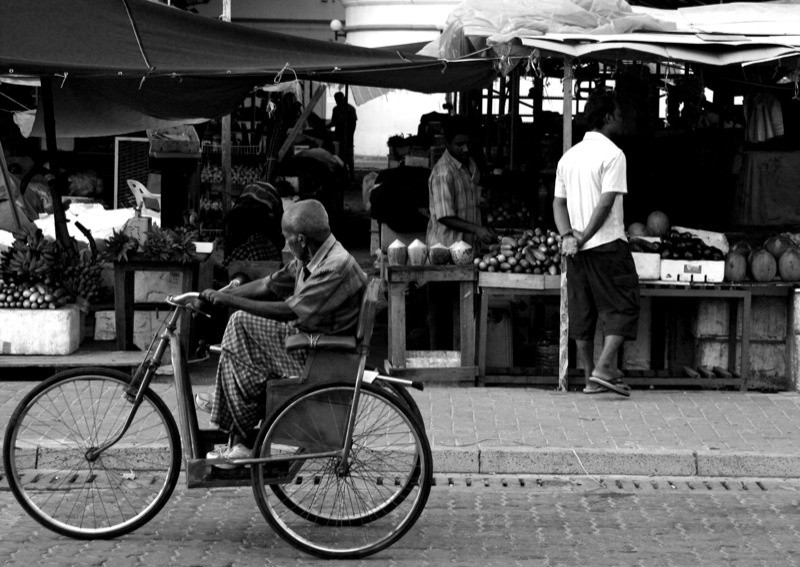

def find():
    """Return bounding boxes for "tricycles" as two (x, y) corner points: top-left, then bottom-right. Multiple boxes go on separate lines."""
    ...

(1, 276), (436, 559)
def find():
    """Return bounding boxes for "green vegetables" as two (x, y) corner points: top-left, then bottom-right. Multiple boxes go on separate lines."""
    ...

(104, 222), (210, 263)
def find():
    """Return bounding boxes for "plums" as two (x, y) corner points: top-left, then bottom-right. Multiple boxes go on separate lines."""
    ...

(653, 230), (725, 261)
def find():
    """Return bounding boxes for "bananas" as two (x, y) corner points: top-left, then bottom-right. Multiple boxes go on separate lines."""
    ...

(0, 233), (102, 299)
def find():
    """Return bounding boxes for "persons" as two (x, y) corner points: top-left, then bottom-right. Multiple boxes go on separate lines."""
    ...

(291, 139), (351, 194)
(186, 199), (367, 469)
(326, 92), (358, 184)
(551, 97), (642, 399)
(425, 123), (497, 246)
(278, 92), (302, 125)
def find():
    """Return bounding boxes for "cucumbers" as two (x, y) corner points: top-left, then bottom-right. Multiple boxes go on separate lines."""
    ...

(473, 228), (561, 276)
(0, 278), (72, 308)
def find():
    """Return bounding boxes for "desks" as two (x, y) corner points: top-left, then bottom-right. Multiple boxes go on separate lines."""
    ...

(387, 265), (481, 387)
(114, 253), (201, 359)
(479, 286), (798, 392)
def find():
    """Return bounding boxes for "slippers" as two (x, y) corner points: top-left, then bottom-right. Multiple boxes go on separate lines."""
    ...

(589, 377), (631, 397)
(584, 386), (607, 392)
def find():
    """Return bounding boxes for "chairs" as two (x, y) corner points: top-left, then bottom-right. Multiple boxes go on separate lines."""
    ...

(260, 279), (383, 452)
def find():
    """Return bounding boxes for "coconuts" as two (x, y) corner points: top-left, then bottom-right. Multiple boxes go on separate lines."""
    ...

(626, 210), (669, 236)
(724, 236), (800, 283)
(387, 239), (474, 266)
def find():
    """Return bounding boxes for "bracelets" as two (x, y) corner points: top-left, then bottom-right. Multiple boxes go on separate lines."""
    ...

(560, 231), (573, 239)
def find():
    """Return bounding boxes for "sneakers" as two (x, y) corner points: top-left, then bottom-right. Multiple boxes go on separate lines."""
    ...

(206, 444), (254, 468)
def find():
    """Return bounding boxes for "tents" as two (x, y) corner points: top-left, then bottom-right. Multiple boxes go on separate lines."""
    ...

(2, 0), (527, 260)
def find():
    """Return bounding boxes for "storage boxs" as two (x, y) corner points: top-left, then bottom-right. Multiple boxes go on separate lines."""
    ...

(381, 223), (427, 252)
(479, 227), (787, 377)
(275, 126), (339, 192)
(0, 121), (272, 353)
(388, 122), (448, 171)
